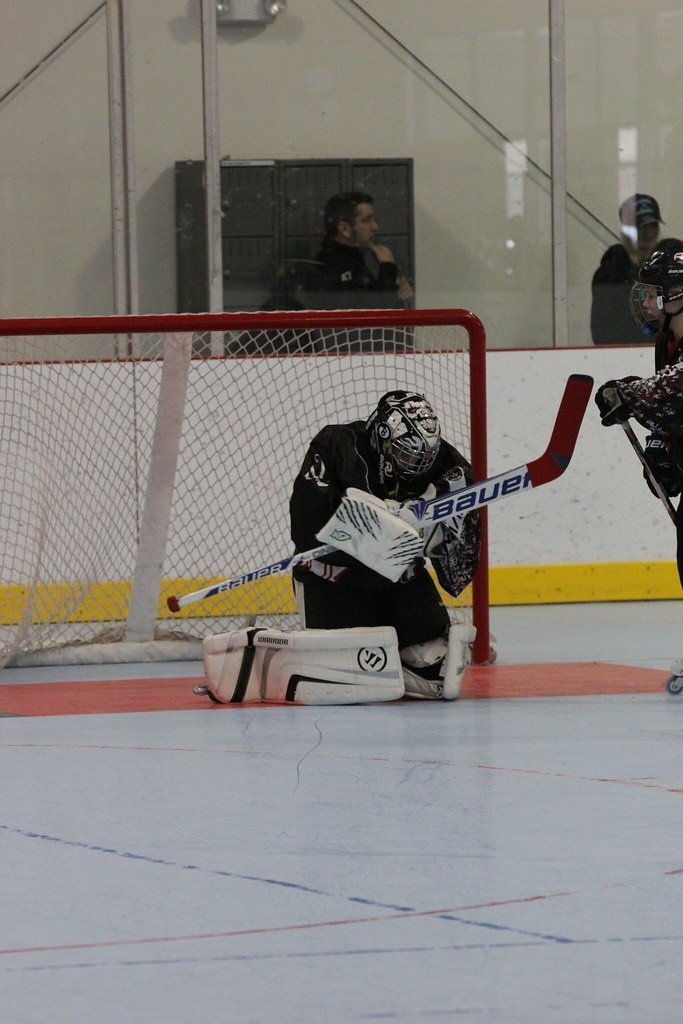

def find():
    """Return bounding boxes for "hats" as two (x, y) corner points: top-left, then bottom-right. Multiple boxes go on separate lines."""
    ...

(620, 194), (666, 229)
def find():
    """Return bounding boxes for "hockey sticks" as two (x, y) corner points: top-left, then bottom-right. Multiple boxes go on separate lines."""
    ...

(165, 372), (595, 613)
(602, 386), (676, 528)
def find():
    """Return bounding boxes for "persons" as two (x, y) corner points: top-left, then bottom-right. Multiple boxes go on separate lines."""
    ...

(590, 193), (666, 346)
(304, 191), (417, 352)
(594, 237), (683, 696)
(192, 390), (485, 706)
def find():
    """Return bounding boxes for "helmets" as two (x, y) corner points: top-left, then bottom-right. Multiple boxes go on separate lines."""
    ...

(362, 390), (441, 487)
(629, 243), (683, 337)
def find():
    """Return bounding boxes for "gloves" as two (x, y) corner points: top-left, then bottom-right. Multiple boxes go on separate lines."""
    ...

(643, 435), (683, 498)
(595, 381), (634, 427)
